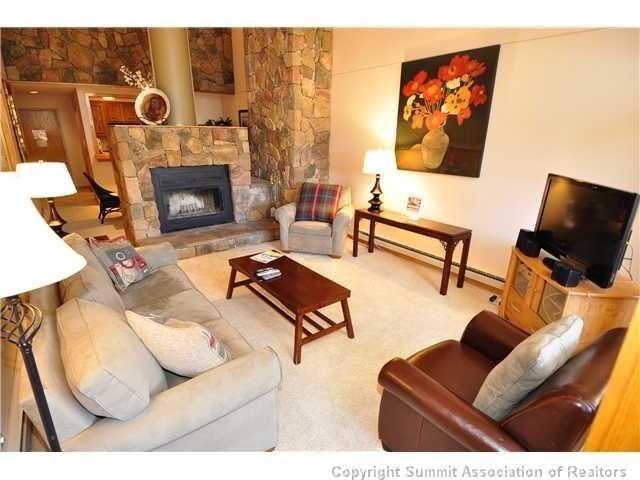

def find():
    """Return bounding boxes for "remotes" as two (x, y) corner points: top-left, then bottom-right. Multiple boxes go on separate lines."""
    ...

(256, 267), (272, 272)
(262, 272), (281, 280)
(256, 269), (280, 277)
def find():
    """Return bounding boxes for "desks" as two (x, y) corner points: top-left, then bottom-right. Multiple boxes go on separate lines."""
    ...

(352, 207), (473, 296)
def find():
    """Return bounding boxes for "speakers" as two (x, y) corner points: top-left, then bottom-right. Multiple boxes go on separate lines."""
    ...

(551, 262), (577, 287)
(515, 228), (541, 257)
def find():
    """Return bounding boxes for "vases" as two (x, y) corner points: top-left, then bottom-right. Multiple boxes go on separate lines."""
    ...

(419, 127), (450, 169)
(134, 86), (170, 125)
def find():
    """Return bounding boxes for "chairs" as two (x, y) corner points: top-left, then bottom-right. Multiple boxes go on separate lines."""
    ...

(274, 182), (356, 260)
(376, 309), (627, 452)
(82, 171), (123, 226)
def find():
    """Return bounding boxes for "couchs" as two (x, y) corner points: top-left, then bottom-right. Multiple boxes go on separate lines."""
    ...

(0, 233), (284, 452)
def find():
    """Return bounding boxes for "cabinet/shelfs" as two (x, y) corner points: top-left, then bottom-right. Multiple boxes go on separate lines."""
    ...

(107, 101), (141, 124)
(89, 100), (110, 138)
(495, 245), (640, 357)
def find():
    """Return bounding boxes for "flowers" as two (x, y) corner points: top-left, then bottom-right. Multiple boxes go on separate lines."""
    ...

(401, 55), (489, 132)
(119, 64), (155, 90)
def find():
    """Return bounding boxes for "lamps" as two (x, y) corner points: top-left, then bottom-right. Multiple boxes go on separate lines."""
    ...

(362, 149), (398, 213)
(1, 171), (89, 453)
(15, 159), (77, 238)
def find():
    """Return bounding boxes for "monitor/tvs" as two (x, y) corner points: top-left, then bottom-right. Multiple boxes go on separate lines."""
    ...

(534, 173), (640, 289)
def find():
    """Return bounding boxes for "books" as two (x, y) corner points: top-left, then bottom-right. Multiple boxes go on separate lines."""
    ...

(249, 249), (285, 265)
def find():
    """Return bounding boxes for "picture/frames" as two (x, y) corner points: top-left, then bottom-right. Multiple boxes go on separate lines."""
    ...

(403, 194), (423, 220)
(237, 109), (249, 127)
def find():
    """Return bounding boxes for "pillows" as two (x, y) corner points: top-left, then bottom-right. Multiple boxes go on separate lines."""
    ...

(295, 183), (342, 224)
(472, 313), (584, 422)
(88, 237), (157, 292)
(123, 311), (232, 378)
